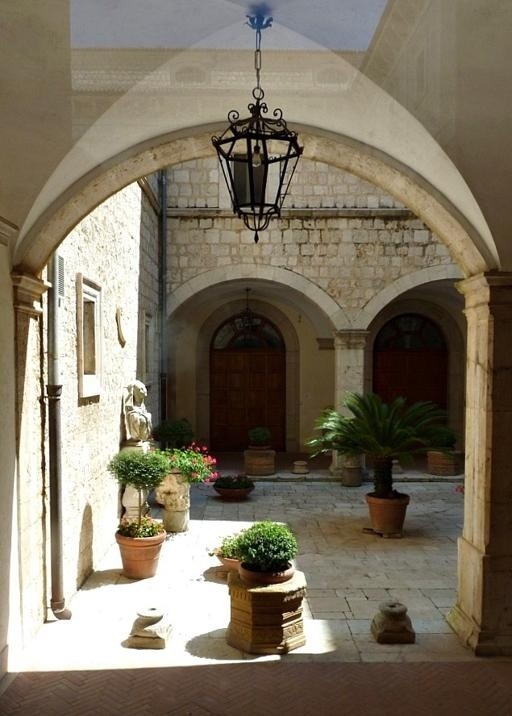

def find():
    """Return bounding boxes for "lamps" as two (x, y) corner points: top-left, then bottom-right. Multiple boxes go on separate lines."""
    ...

(211, 13), (303, 244)
(240, 288), (254, 330)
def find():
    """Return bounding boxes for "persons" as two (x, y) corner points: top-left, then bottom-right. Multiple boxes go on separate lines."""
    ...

(121, 379), (154, 441)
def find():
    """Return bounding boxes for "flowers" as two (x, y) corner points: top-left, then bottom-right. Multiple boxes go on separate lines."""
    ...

(156, 443), (217, 484)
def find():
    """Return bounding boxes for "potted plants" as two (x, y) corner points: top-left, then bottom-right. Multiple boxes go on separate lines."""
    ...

(107, 450), (170, 579)
(427, 424), (463, 476)
(207, 521), (308, 655)
(245, 428), (274, 475)
(213, 475), (255, 499)
(304, 391), (458, 535)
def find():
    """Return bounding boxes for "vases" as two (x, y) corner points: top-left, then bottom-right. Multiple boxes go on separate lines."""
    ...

(161, 468), (190, 532)
